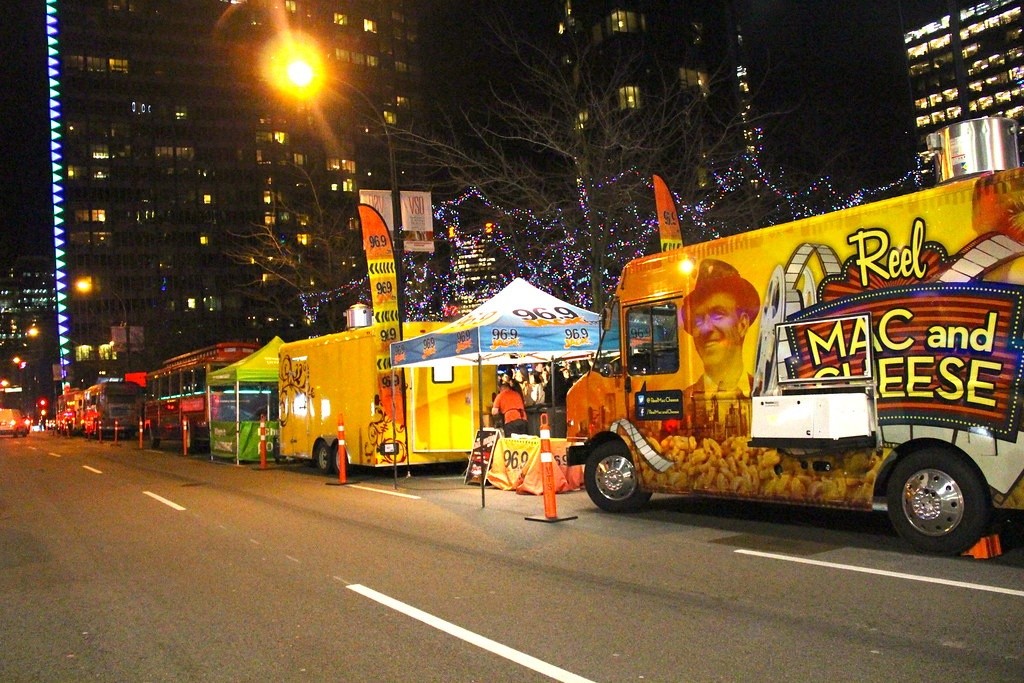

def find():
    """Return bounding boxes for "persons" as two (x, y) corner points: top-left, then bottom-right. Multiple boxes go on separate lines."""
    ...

(659, 256), (761, 439)
(490, 360), (590, 438)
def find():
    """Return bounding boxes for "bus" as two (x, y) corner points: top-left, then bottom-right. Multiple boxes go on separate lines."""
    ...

(54, 381), (145, 438)
(143, 340), (262, 455)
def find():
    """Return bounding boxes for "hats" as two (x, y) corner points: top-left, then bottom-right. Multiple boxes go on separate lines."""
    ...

(505, 368), (513, 378)
(680, 258), (760, 336)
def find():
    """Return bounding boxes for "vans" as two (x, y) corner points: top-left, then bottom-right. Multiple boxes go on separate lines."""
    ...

(0, 409), (29, 439)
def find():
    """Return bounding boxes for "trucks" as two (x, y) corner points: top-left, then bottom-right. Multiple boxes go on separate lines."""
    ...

(560, 113), (1024, 555)
(279, 304), (501, 476)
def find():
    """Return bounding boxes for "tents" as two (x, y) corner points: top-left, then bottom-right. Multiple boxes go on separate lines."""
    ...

(389, 276), (663, 490)
(205, 336), (286, 466)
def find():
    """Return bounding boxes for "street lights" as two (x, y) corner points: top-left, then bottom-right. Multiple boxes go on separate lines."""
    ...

(77, 280), (131, 373)
(288, 59), (408, 325)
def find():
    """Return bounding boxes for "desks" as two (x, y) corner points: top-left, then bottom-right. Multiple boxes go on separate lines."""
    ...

(492, 438), (583, 496)
